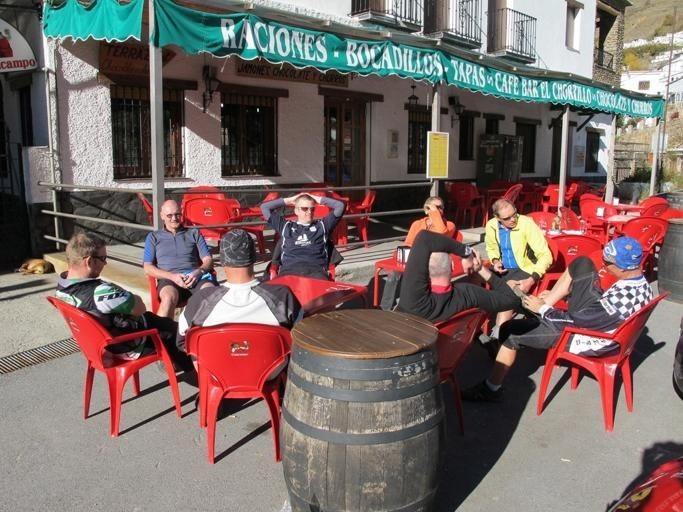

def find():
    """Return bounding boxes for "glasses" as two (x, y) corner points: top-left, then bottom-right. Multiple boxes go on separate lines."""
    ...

(83, 255), (107, 263)
(298, 206), (314, 211)
(601, 257), (613, 266)
(500, 209), (517, 221)
(164, 213), (180, 218)
(437, 205), (444, 209)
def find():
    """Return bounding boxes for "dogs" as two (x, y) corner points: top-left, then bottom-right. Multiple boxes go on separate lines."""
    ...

(15, 257), (55, 275)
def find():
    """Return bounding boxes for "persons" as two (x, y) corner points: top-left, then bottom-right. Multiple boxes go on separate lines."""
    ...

(460, 237), (654, 402)
(143, 201), (216, 320)
(484, 198), (553, 339)
(394, 229), (522, 325)
(380, 196), (456, 310)
(175, 228), (305, 383)
(260, 192), (346, 281)
(55, 231), (194, 372)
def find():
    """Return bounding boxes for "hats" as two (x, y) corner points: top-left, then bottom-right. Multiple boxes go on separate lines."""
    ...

(603, 238), (642, 270)
(220, 230), (257, 267)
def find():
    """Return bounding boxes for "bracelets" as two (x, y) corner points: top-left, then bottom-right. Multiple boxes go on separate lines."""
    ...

(199, 268), (206, 274)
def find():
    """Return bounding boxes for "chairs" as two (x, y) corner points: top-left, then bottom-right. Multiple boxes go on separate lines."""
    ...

(270, 262), (335, 282)
(185, 322), (292, 464)
(46, 295), (182, 437)
(137, 183), (376, 254)
(148, 262), (218, 317)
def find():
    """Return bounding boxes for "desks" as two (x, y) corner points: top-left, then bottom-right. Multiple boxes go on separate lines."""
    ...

(264, 275), (368, 318)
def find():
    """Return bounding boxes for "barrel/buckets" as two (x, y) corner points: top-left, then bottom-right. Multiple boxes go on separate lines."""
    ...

(279, 310), (447, 512)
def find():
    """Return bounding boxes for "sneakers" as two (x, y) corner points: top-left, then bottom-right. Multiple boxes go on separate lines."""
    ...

(478, 332), (498, 358)
(461, 379), (504, 403)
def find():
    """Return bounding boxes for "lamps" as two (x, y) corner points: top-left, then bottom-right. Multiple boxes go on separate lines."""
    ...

(451, 101), (466, 127)
(408, 81), (419, 112)
(201, 76), (221, 113)
